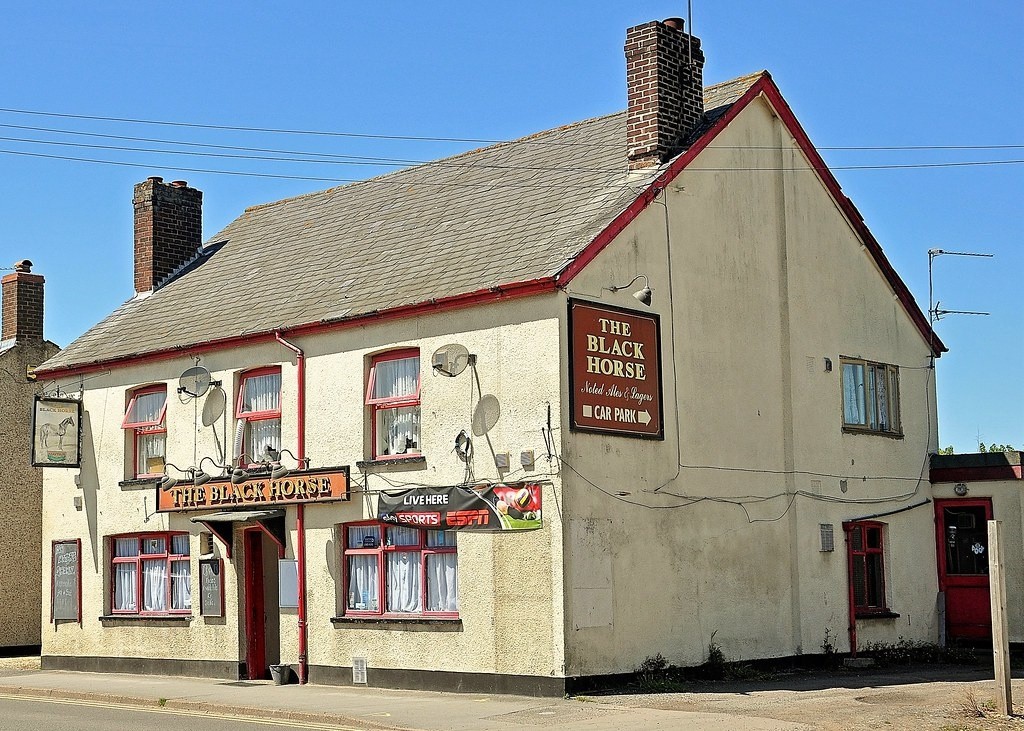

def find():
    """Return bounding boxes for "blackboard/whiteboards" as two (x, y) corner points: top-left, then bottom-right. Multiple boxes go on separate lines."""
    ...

(52, 542), (82, 621)
(200, 560), (221, 618)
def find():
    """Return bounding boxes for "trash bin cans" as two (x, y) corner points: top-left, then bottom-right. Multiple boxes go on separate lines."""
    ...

(269, 665), (291, 686)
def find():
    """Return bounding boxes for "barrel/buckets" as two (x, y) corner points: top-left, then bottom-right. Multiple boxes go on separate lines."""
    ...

(269, 665), (290, 684)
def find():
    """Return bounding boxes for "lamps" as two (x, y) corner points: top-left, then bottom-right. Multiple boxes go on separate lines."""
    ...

(161, 463), (196, 492)
(198, 531), (213, 556)
(269, 449), (310, 480)
(611, 274), (654, 307)
(947, 521), (959, 549)
(231, 454), (269, 485)
(195, 457), (232, 486)
